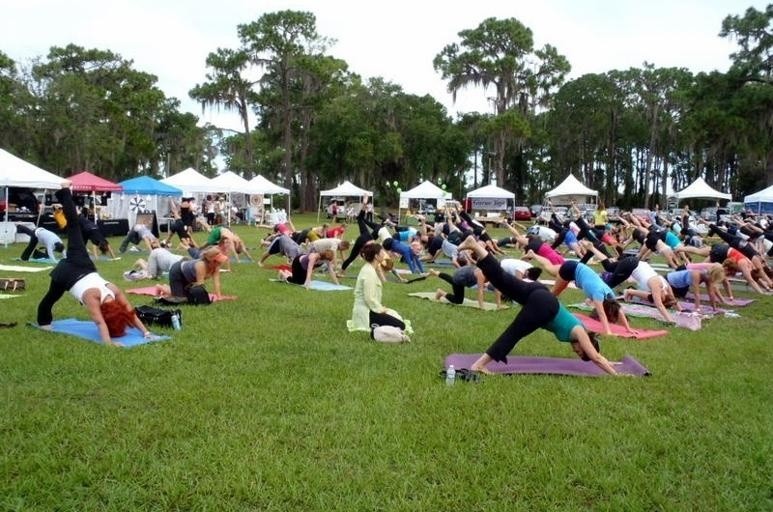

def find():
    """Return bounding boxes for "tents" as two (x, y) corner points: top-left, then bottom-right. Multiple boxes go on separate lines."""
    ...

(0, 147), (183, 248)
(543, 173), (599, 227)
(465, 184), (515, 228)
(744, 186), (772, 216)
(317, 180), (374, 225)
(160, 167), (290, 228)
(398, 181), (452, 228)
(674, 177), (732, 215)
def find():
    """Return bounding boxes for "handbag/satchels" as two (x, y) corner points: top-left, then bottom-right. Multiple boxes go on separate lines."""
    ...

(374, 325), (403, 343)
(33, 249), (49, 259)
(186, 286), (210, 305)
(461, 369), (478, 381)
(0, 277), (26, 291)
(134, 305), (181, 328)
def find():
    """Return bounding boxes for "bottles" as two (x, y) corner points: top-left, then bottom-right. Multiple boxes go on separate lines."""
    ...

(725, 313), (740, 319)
(170, 314), (180, 330)
(446, 364), (456, 385)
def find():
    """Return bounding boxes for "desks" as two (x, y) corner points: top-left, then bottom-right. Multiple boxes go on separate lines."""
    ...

(95, 218), (129, 237)
(3, 221), (37, 243)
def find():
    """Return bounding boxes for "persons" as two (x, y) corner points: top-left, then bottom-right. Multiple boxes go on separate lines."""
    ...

(257, 195), (772, 376)
(3, 180), (248, 351)
